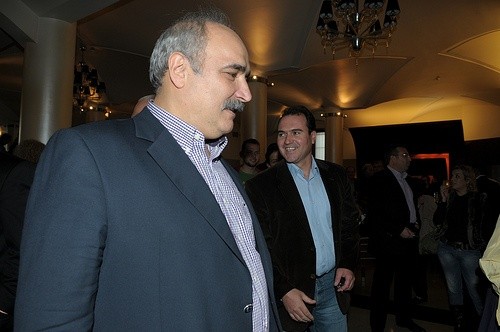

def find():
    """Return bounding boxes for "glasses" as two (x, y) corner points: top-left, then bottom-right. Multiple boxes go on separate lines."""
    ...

(397, 153), (409, 158)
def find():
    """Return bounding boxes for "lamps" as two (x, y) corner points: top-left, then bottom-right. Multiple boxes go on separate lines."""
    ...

(316, 0), (401, 57)
(73, 41), (112, 117)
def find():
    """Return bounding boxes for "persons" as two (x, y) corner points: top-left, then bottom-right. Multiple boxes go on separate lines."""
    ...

(13, 6), (282, 332)
(361, 145), (425, 332)
(412, 177), (455, 304)
(346, 158), (385, 194)
(433, 165), (487, 332)
(131, 95), (155, 118)
(474, 159), (500, 233)
(265, 143), (285, 168)
(478, 212), (500, 332)
(233, 139), (262, 191)
(245, 106), (360, 332)
